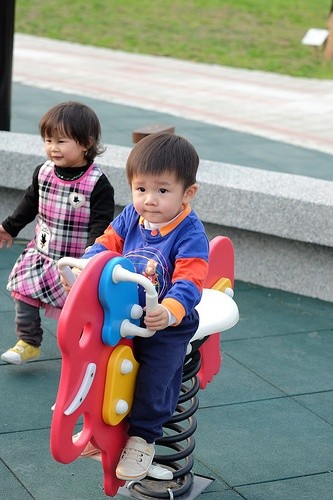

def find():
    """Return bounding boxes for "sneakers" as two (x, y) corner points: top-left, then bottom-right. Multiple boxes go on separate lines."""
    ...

(116, 436), (155, 480)
(72, 431), (100, 458)
(1, 339), (41, 365)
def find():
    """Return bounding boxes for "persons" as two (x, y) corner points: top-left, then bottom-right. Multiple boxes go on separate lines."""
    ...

(0, 100), (116, 365)
(57, 130), (211, 482)
